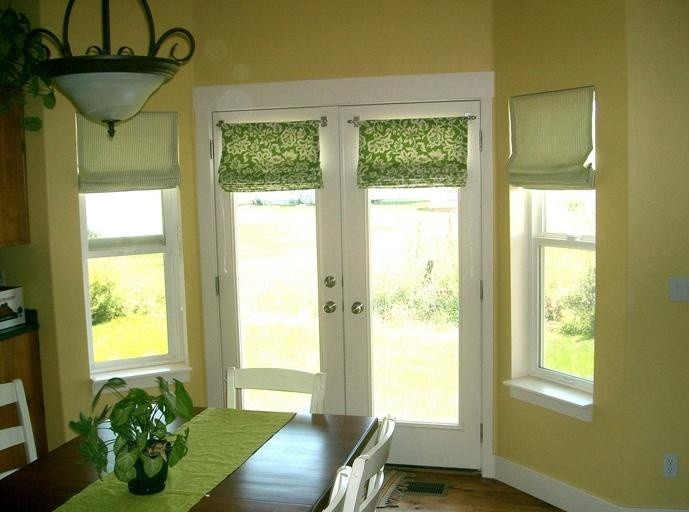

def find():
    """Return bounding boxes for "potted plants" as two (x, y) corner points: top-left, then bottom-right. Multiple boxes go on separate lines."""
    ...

(70, 376), (200, 495)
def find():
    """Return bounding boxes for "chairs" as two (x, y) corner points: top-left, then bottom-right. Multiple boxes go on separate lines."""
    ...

(0, 377), (36, 484)
(222, 366), (326, 415)
(329, 419), (400, 512)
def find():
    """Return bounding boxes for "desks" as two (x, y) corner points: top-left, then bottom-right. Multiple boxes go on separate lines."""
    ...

(0, 406), (378, 509)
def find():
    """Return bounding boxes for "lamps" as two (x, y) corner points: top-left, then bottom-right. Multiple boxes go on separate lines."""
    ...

(19, 0), (197, 141)
